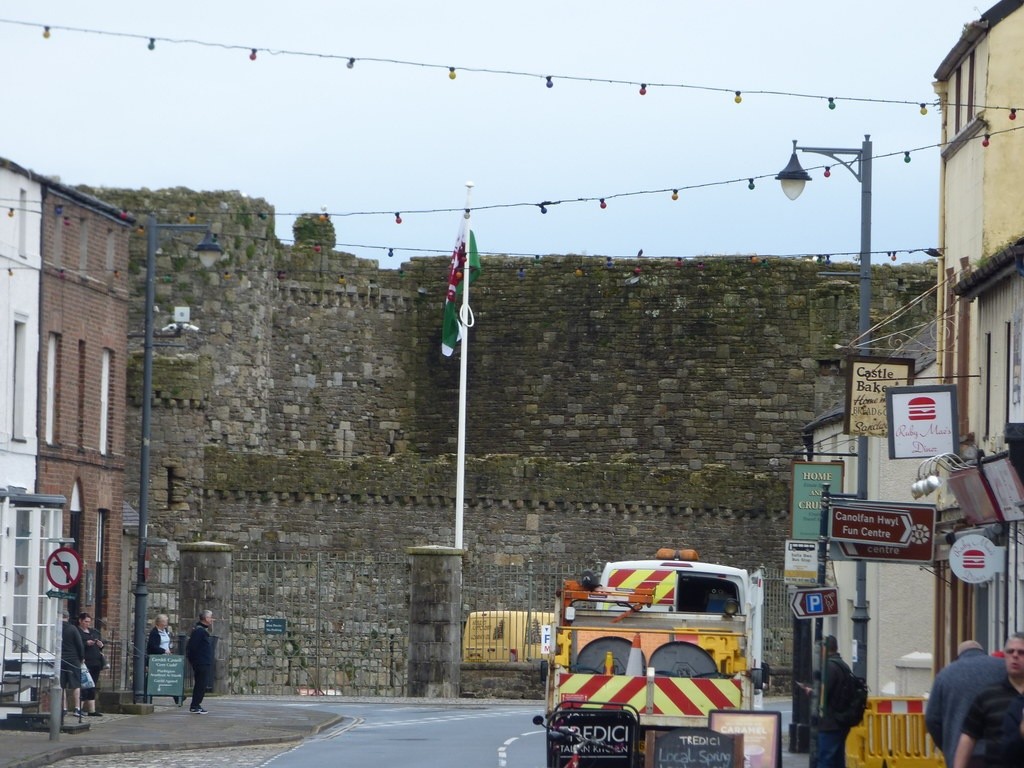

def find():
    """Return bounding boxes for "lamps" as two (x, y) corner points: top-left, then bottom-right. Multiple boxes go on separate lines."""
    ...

(913, 452), (977, 500)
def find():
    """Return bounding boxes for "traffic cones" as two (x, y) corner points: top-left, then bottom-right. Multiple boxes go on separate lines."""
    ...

(625, 630), (645, 677)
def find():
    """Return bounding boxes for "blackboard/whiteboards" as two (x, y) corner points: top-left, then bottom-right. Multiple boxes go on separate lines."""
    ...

(546, 709), (636, 768)
(147, 654), (185, 696)
(655, 727), (733, 768)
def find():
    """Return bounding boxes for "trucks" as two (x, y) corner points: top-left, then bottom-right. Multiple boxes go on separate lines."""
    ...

(545, 550), (770, 715)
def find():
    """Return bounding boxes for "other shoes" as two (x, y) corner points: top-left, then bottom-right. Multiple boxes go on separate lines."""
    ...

(175, 696), (186, 704)
(88, 712), (102, 715)
(63, 709), (67, 717)
(74, 707), (84, 718)
(198, 707), (208, 715)
(190, 707), (200, 713)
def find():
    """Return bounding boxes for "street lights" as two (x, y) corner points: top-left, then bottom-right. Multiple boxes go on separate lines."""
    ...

(121, 212), (226, 714)
(774, 135), (872, 680)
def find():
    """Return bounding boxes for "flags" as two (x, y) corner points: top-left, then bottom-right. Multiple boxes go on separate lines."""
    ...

(442, 204), (482, 357)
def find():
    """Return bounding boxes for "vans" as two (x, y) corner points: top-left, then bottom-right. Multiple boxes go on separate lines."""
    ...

(461, 611), (555, 663)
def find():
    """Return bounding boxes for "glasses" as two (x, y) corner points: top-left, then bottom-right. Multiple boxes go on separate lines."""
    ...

(206, 616), (212, 619)
(1006, 649), (1024, 656)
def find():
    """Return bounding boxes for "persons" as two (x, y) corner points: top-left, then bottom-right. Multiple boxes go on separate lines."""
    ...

(147, 614), (186, 703)
(77, 613), (104, 716)
(925, 640), (1008, 768)
(186, 610), (212, 714)
(805, 634), (851, 768)
(953, 632), (1024, 768)
(60, 611), (84, 717)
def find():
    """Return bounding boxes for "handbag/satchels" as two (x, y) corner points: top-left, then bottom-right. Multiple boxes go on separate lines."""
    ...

(80, 663), (95, 689)
(102, 656), (110, 668)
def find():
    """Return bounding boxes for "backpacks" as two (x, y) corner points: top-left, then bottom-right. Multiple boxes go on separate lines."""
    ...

(827, 658), (868, 728)
